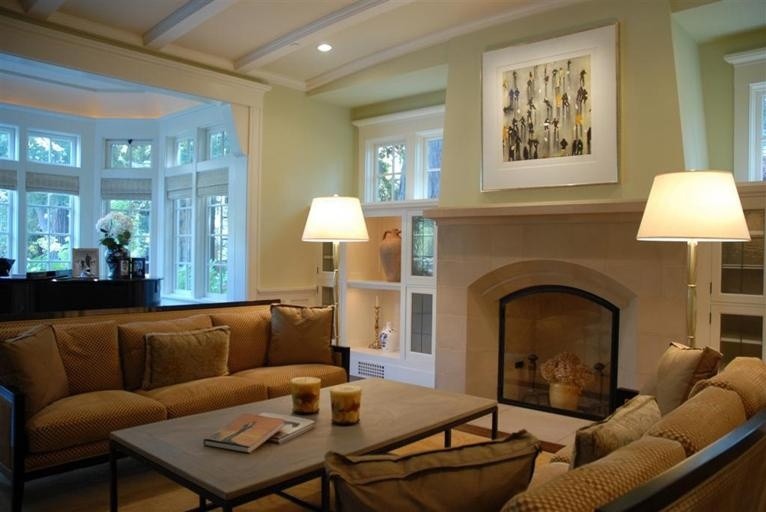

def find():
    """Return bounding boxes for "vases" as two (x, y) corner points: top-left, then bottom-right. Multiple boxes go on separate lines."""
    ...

(105, 247), (131, 278)
(548, 381), (579, 410)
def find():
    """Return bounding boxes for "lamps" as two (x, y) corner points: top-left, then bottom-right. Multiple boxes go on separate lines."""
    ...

(635, 169), (752, 349)
(298, 197), (368, 346)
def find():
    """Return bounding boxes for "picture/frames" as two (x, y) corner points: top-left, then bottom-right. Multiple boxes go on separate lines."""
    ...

(480, 22), (618, 192)
(133, 257), (145, 276)
(118, 258), (130, 276)
(72, 248), (98, 279)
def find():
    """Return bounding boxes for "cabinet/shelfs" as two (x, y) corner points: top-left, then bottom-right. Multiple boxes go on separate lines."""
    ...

(338, 200), (437, 387)
(695, 181), (766, 358)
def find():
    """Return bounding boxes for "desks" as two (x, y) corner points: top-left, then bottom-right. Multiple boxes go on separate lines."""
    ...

(0, 271), (164, 310)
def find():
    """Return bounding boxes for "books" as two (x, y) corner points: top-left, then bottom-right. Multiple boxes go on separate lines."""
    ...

(203, 412), (285, 454)
(262, 412), (315, 444)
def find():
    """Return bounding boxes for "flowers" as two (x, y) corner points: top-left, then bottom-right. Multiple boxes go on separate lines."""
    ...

(95, 210), (132, 247)
(540, 351), (595, 395)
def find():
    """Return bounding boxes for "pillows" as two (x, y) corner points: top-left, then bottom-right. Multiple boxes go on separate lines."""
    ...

(138, 325), (231, 390)
(567, 391), (663, 468)
(322, 429), (538, 511)
(646, 342), (722, 420)
(267, 301), (335, 364)
(6, 323), (69, 417)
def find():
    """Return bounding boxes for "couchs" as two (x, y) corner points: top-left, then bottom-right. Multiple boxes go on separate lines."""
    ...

(0, 299), (351, 512)
(498, 354), (766, 512)
